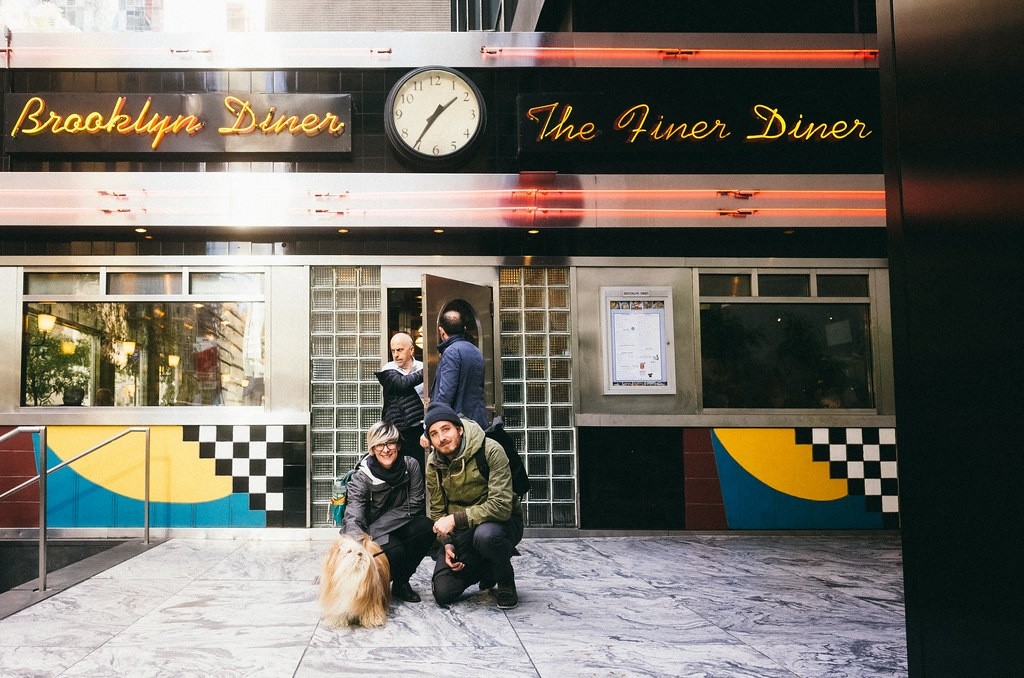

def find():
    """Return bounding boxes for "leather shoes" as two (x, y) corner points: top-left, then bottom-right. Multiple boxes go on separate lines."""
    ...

(392, 581), (421, 602)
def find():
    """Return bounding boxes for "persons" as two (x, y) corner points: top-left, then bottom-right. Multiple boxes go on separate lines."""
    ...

(420, 310), (493, 449)
(69, 387), (85, 406)
(344, 421), (436, 602)
(376, 332), (428, 472)
(96, 389), (113, 406)
(426, 401), (524, 609)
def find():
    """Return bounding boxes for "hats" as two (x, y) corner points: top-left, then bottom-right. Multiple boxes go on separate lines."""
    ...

(424, 402), (465, 438)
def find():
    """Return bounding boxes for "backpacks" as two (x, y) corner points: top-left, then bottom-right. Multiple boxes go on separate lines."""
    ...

(475, 417), (531, 497)
(332, 454), (368, 535)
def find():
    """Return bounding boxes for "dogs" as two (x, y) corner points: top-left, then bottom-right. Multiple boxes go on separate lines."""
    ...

(314, 534), (391, 627)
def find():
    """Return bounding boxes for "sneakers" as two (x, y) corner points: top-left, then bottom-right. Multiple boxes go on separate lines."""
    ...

(496, 576), (519, 609)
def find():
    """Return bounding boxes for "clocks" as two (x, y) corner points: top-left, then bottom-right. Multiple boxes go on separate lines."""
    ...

(384, 65), (489, 168)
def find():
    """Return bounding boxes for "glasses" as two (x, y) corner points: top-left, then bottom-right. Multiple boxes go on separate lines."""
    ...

(374, 442), (399, 452)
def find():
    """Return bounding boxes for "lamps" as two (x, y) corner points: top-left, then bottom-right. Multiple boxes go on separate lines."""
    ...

(26, 314), (56, 349)
